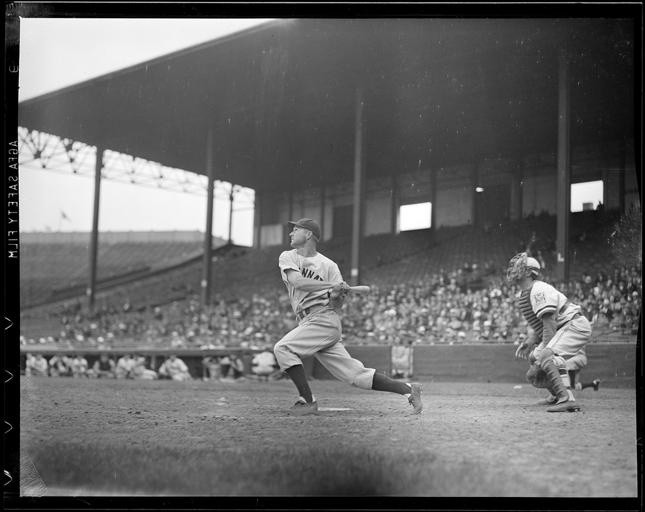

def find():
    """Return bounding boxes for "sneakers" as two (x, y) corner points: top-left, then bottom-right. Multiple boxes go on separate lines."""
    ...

(285, 398), (318, 415)
(591, 379), (600, 391)
(535, 394), (582, 413)
(408, 382), (424, 416)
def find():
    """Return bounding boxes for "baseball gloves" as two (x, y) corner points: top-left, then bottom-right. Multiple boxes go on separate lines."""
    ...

(526, 361), (550, 388)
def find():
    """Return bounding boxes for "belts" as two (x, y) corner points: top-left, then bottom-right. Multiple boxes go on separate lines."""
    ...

(296, 308), (311, 320)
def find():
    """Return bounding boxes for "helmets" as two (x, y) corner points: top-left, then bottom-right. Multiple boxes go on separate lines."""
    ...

(506, 251), (540, 280)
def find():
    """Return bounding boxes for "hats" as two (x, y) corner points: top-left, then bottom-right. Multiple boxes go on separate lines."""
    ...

(288, 217), (321, 242)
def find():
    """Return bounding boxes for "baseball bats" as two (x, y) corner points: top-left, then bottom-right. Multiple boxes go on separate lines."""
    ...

(341, 284), (373, 296)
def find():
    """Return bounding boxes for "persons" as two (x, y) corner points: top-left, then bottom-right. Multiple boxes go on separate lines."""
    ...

(506, 252), (591, 414)
(20, 277), (641, 382)
(432, 197), (645, 277)
(566, 344), (601, 392)
(272, 217), (422, 415)
